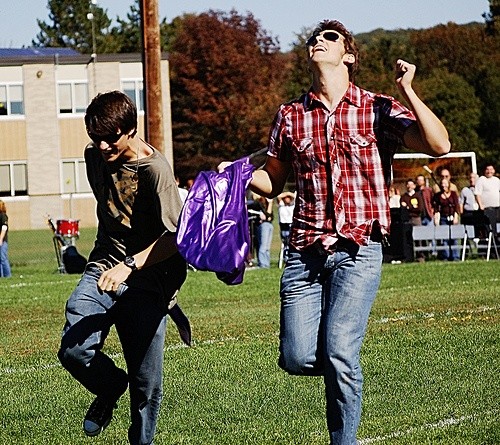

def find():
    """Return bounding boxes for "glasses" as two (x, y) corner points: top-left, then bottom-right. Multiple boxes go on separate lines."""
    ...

(440, 176), (449, 179)
(88, 131), (127, 143)
(305, 33), (348, 53)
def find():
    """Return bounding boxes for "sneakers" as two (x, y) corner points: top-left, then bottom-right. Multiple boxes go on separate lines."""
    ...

(82, 369), (129, 435)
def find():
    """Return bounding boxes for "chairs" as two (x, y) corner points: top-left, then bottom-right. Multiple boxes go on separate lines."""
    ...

(410, 222), (500, 262)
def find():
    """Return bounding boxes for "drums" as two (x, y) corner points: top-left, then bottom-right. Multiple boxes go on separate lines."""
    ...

(55, 219), (79, 235)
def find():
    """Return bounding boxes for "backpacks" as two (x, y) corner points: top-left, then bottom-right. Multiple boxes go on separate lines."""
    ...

(62, 245), (87, 274)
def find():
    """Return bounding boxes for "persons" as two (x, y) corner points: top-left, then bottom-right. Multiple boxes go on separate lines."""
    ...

(58, 90), (192, 445)
(277, 191), (296, 262)
(389, 165), (500, 262)
(218, 20), (450, 445)
(174, 170), (196, 272)
(254, 197), (273, 268)
(0, 199), (12, 277)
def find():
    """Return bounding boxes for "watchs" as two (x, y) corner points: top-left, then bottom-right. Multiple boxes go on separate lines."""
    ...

(124, 255), (139, 272)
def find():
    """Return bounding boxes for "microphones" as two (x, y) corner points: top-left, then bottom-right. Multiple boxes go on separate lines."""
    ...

(421, 164), (434, 175)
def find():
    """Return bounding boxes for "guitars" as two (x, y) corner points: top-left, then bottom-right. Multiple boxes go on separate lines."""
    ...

(46, 215), (89, 274)
